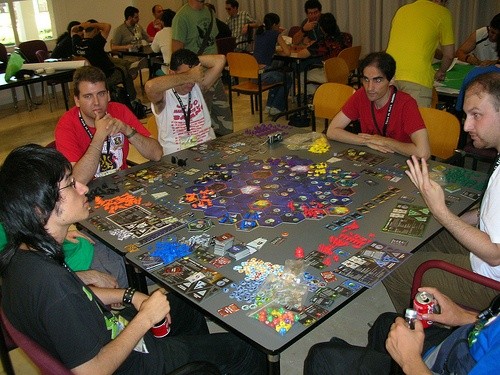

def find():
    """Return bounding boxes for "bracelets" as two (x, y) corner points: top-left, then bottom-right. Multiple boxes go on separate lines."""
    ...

(127, 126), (137, 139)
(123, 287), (136, 304)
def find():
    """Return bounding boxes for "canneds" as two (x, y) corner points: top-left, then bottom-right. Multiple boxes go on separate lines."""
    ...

(413, 290), (434, 328)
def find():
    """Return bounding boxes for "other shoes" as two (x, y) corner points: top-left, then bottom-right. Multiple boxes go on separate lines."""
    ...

(263, 106), (281, 116)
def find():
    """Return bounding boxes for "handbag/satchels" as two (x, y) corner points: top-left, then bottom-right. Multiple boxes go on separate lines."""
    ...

(289, 113), (311, 128)
(128, 98), (147, 119)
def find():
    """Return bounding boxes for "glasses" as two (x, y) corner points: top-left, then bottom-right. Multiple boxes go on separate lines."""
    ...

(59, 177), (76, 191)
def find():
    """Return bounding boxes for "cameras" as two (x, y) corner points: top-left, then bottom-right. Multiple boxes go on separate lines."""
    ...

(268, 131), (283, 144)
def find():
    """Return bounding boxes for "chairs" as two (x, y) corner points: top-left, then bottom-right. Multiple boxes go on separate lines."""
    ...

(226, 26), (362, 132)
(420, 108), (460, 159)
(0, 40), (56, 107)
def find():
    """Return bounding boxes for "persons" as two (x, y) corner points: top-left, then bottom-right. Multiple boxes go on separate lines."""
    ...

(51, 0), (343, 157)
(303, 0), (500, 375)
(55, 65), (163, 185)
(0, 143), (271, 375)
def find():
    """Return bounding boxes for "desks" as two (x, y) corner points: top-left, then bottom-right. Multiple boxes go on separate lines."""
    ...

(73, 122), (490, 375)
(0, 69), (76, 111)
(109, 45), (162, 86)
(272, 54), (324, 131)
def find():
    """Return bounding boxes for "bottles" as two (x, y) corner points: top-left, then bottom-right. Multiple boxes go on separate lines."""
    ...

(150, 317), (172, 338)
(393, 307), (418, 375)
